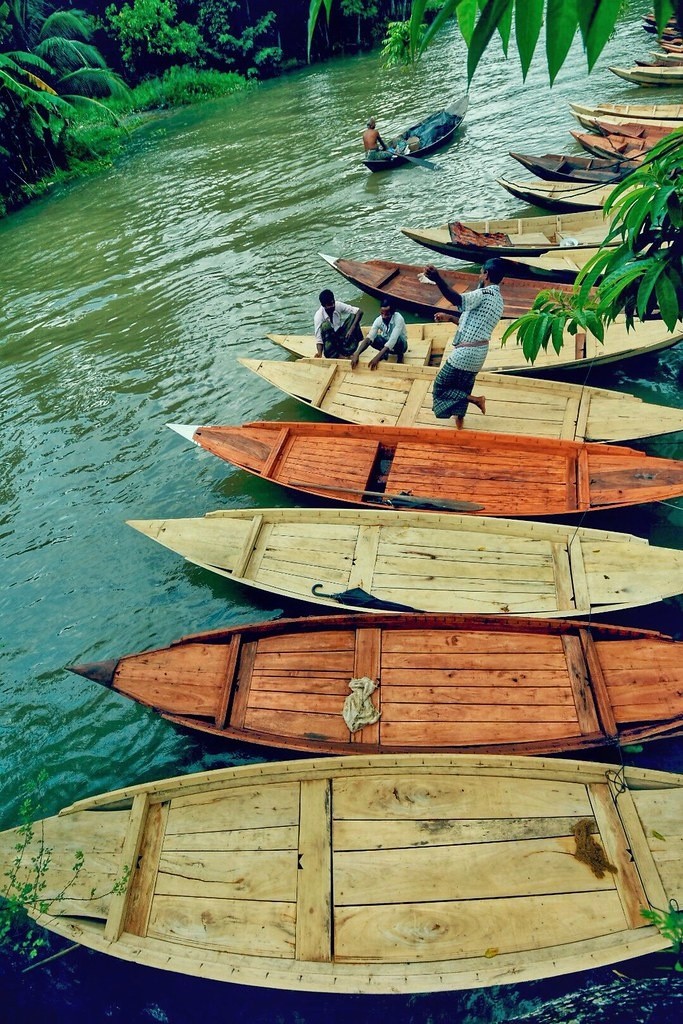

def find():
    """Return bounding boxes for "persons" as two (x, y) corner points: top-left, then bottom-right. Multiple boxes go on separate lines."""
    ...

(351, 298), (408, 370)
(313, 289), (364, 359)
(424, 258), (506, 431)
(362, 118), (387, 159)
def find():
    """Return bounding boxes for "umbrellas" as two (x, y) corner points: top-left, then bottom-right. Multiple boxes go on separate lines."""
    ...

(312, 583), (426, 614)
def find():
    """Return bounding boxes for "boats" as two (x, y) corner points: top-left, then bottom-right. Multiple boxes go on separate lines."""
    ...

(360, 96), (469, 172)
(166, 423), (683, 518)
(125, 509), (683, 616)
(0, 753), (683, 993)
(266, 314), (683, 374)
(236, 356), (683, 443)
(399, 13), (683, 264)
(60, 613), (683, 755)
(318, 252), (640, 318)
